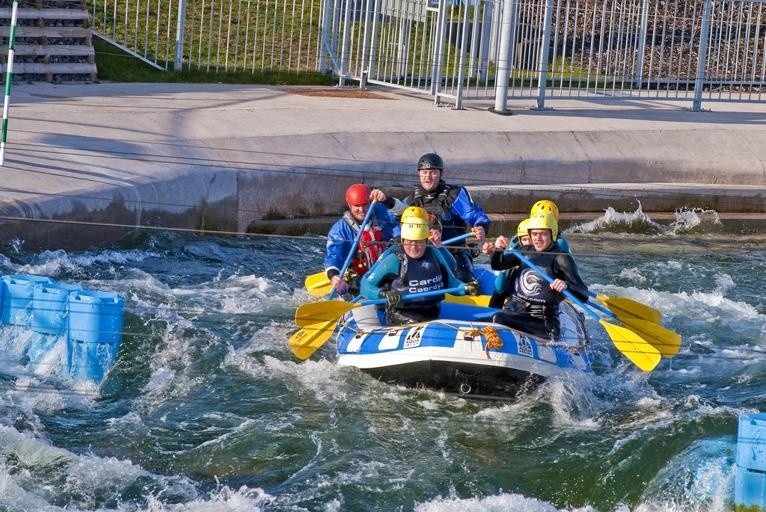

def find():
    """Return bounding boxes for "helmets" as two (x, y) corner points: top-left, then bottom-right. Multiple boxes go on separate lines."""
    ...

(401, 206), (429, 224)
(527, 213), (559, 243)
(530, 199), (559, 221)
(517, 219), (530, 246)
(428, 214), (442, 232)
(418, 153), (443, 170)
(346, 184), (371, 205)
(401, 217), (429, 240)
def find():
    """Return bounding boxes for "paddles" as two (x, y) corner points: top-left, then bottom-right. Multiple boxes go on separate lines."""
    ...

(286, 296), (361, 361)
(305, 231), (476, 297)
(572, 285), (662, 325)
(587, 300), (682, 359)
(294, 285), (474, 327)
(505, 244), (662, 372)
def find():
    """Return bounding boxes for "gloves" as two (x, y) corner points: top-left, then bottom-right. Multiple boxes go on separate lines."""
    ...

(383, 290), (402, 306)
(469, 279), (481, 298)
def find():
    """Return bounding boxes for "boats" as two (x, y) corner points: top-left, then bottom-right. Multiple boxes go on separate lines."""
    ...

(333, 264), (598, 399)
